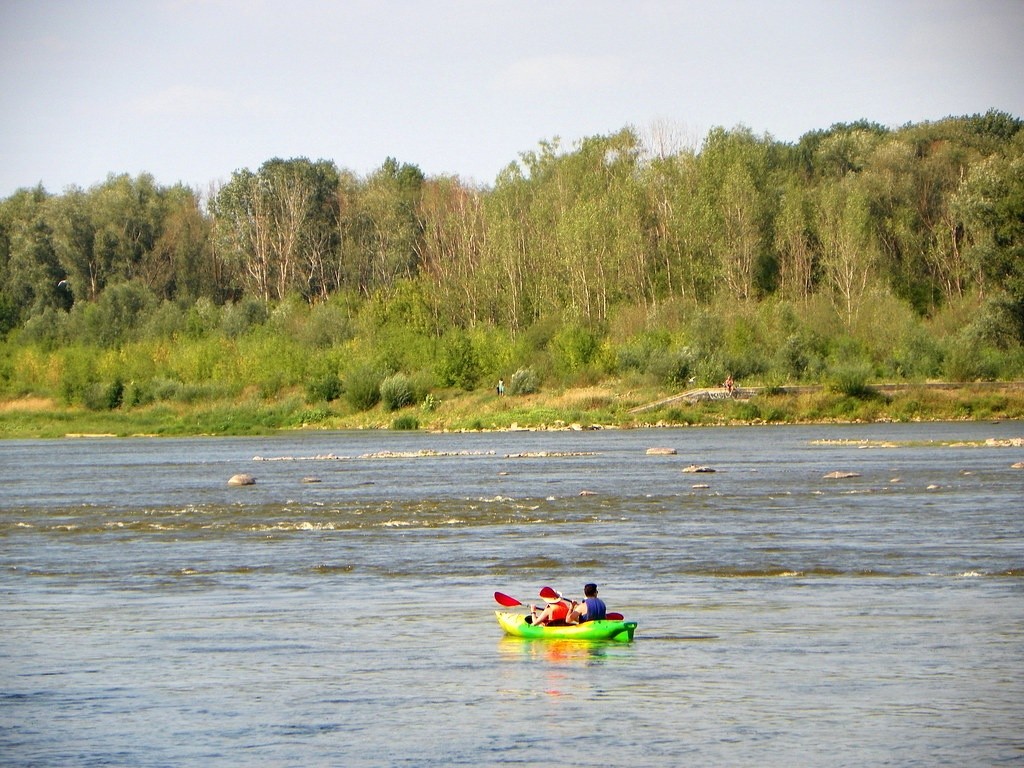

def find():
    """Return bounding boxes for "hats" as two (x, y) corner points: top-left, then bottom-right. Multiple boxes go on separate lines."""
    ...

(543, 590), (562, 603)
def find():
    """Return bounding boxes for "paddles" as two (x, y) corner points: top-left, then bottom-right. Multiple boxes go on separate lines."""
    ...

(494, 591), (548, 612)
(539, 586), (624, 621)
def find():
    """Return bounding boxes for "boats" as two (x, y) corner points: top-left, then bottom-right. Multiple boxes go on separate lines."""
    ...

(495, 608), (638, 643)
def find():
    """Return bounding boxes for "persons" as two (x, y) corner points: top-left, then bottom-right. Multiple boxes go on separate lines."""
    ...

(565, 583), (606, 624)
(496, 378), (505, 396)
(530, 587), (569, 627)
(725, 375), (734, 398)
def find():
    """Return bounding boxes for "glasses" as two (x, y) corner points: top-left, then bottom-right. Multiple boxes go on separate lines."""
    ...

(596, 590), (598, 594)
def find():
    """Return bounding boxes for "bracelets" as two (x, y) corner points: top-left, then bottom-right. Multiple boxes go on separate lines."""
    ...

(531, 612), (536, 614)
